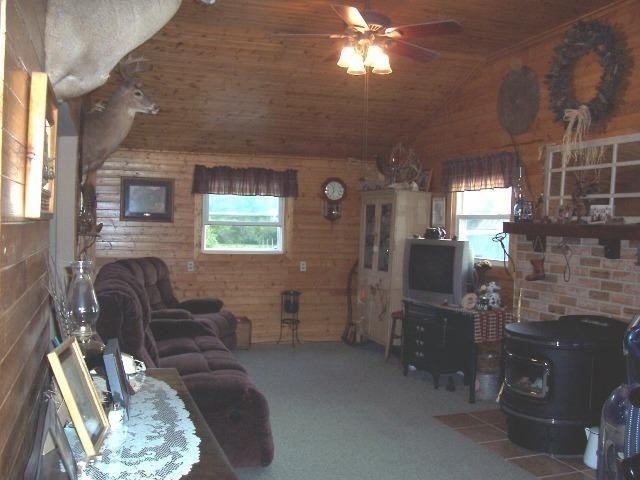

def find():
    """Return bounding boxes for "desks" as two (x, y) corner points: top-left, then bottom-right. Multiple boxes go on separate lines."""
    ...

(38, 367), (243, 480)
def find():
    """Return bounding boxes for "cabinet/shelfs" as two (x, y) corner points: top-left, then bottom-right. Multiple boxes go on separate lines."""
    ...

(356, 190), (431, 347)
(401, 298), (472, 389)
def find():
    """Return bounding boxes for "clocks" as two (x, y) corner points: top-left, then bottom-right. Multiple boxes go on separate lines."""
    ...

(320, 176), (347, 221)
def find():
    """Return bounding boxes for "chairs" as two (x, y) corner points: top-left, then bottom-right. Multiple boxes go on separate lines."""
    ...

(116, 257), (237, 351)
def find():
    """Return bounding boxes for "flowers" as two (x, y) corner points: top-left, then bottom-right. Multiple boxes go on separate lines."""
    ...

(541, 20), (625, 132)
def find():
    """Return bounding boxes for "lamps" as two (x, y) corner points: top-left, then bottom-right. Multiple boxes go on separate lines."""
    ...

(65, 261), (106, 371)
(335, 38), (393, 76)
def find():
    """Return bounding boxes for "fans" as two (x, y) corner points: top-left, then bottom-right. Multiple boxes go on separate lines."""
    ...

(268, 0), (464, 76)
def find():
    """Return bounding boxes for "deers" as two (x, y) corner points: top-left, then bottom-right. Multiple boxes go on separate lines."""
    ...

(78, 52), (161, 175)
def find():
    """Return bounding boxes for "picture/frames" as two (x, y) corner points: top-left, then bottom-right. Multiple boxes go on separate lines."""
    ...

(23, 66), (60, 221)
(418, 168), (433, 192)
(46, 335), (131, 480)
(119, 176), (175, 222)
(432, 195), (447, 227)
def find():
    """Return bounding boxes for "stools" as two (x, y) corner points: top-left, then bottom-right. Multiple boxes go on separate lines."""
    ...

(384, 310), (402, 361)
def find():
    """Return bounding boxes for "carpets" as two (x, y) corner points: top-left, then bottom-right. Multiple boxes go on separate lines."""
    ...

(433, 410), (597, 480)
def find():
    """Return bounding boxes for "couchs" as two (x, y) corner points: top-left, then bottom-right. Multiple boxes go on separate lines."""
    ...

(93, 262), (275, 468)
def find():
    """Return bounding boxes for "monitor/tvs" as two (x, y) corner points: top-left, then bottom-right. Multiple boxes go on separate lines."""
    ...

(124, 180), (171, 219)
(401, 237), (474, 308)
